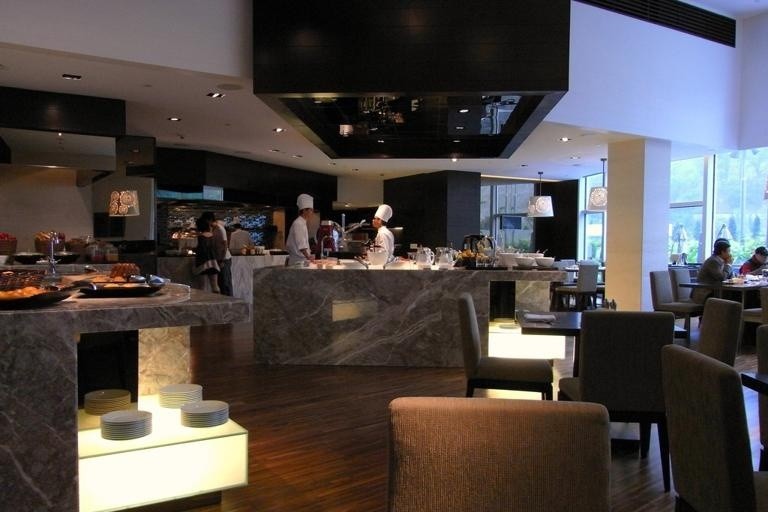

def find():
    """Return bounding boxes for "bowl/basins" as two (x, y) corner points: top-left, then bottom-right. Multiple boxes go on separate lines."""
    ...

(13, 251), (81, 264)
(499, 252), (555, 267)
(365, 250), (393, 267)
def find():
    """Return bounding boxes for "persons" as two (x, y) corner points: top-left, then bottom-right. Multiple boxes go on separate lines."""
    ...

(195, 218), (221, 294)
(229, 216), (253, 250)
(372, 204), (394, 259)
(286, 194), (316, 265)
(714, 238), (729, 248)
(200, 212), (233, 296)
(739, 246), (768, 276)
(689, 241), (734, 303)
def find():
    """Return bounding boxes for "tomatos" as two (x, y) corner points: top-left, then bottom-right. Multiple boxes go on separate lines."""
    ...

(0, 233), (16, 240)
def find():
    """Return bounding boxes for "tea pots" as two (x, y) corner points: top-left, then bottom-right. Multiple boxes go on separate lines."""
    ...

(416, 244), (458, 268)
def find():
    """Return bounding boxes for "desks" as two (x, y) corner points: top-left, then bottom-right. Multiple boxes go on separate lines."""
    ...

(679, 277), (767, 308)
(514, 310), (687, 452)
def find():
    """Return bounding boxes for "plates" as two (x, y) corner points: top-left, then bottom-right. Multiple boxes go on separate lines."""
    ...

(340, 258), (369, 268)
(157, 381), (205, 406)
(83, 387), (131, 415)
(180, 400), (230, 426)
(1, 274), (164, 309)
(99, 408), (153, 439)
(384, 260), (416, 269)
(721, 274), (761, 284)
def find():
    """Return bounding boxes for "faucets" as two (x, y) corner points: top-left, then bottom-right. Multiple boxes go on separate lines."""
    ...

(320, 235), (332, 258)
(48, 230), (63, 265)
(354, 254), (369, 269)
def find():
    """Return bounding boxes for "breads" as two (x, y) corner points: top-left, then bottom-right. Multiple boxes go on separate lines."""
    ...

(458, 250), (475, 257)
(37, 232), (52, 241)
(476, 253), (490, 262)
(110, 263), (140, 278)
(104, 282), (146, 287)
(0, 287), (49, 299)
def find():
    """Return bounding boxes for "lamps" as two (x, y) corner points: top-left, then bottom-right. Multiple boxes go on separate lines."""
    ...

(588, 157), (608, 212)
(526, 172), (554, 217)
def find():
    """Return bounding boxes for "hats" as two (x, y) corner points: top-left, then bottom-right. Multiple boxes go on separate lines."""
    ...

(296, 193), (314, 211)
(375, 204), (393, 223)
(233, 216), (240, 224)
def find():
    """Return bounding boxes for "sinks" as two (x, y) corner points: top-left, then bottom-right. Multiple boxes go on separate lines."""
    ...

(14, 264), (81, 274)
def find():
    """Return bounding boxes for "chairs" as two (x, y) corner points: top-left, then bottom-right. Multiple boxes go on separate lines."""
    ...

(387, 396), (611, 512)
(551, 252), (704, 303)
(457, 292), (554, 402)
(554, 261), (599, 309)
(557, 310), (675, 494)
(661, 344), (767, 511)
(687, 297), (744, 368)
(650, 270), (702, 346)
(738, 286), (767, 354)
(670, 266), (704, 329)
